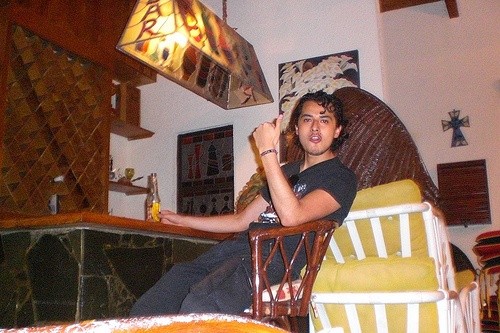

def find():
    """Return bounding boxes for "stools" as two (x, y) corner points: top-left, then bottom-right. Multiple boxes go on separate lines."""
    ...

(479, 264), (500, 321)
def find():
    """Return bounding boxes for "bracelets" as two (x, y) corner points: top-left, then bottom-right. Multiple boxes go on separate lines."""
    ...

(259, 149), (277, 156)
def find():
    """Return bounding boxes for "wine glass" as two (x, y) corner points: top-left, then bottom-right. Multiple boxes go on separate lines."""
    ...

(125, 169), (134, 185)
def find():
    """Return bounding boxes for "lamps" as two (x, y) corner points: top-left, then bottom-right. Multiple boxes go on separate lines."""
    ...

(116, 0), (274, 110)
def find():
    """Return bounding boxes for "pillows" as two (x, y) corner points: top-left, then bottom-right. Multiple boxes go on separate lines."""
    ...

(472, 231), (500, 266)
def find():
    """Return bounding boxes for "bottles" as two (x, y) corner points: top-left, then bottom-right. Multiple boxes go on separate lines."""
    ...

(147, 173), (160, 222)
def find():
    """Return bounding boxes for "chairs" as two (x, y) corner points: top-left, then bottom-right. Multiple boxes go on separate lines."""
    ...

(239, 220), (339, 333)
(300, 180), (480, 333)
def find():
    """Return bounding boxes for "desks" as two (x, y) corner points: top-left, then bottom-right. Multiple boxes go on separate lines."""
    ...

(0, 212), (236, 330)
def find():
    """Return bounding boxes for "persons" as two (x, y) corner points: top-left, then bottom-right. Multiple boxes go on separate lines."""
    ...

(129, 89), (356, 317)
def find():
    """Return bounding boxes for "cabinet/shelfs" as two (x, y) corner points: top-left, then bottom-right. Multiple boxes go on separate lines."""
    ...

(108, 116), (156, 196)
